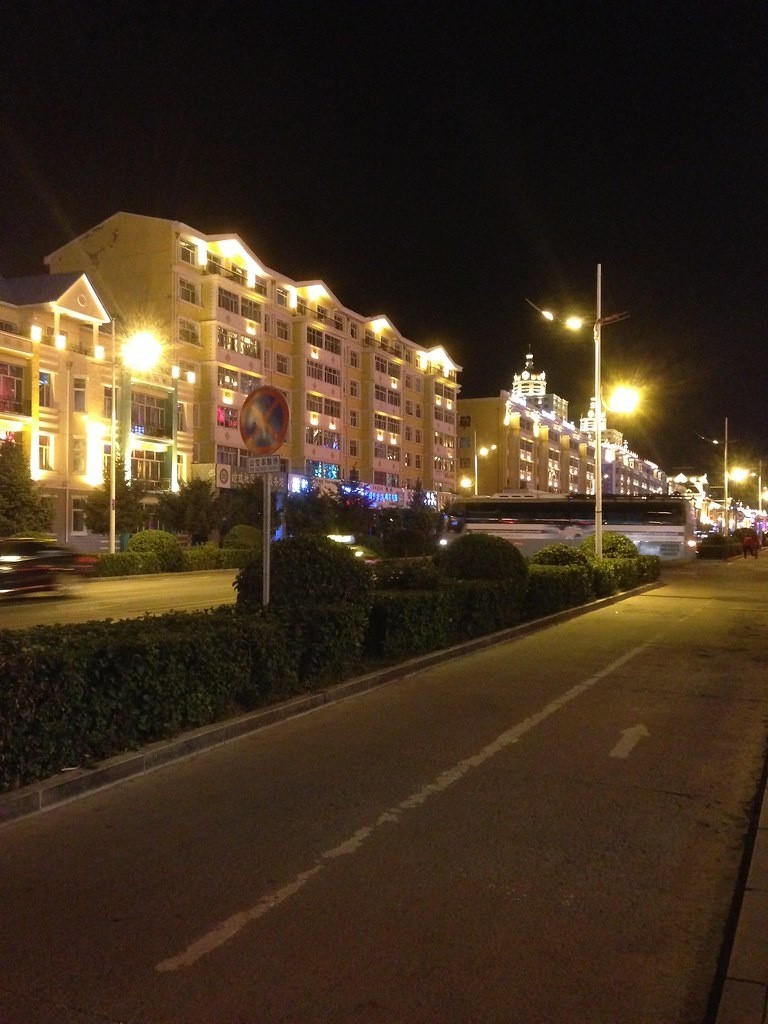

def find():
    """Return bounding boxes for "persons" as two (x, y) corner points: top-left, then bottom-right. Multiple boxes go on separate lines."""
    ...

(744, 535), (756, 558)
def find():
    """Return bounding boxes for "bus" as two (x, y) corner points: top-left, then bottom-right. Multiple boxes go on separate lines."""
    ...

(445, 489), (694, 562)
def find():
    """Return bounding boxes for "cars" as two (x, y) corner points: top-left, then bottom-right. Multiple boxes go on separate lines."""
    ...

(0, 535), (96, 599)
(327, 534), (381, 564)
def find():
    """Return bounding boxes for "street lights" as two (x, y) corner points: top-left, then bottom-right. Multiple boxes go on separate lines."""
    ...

(473, 431), (496, 496)
(695, 417), (738, 537)
(525, 264), (630, 560)
(110, 317), (161, 554)
(733, 458), (762, 538)
